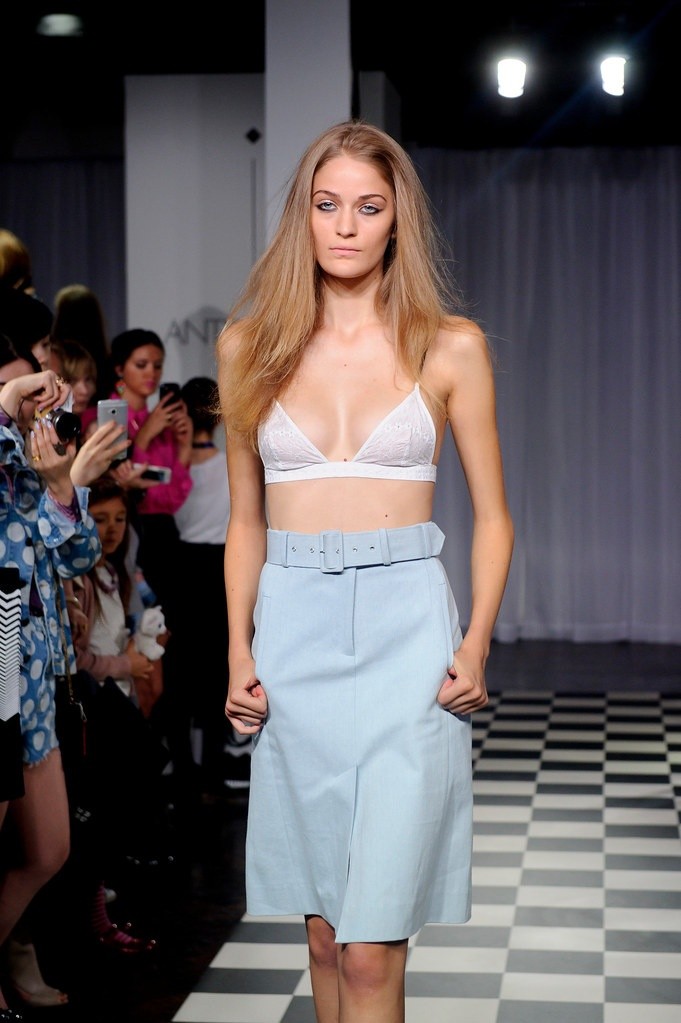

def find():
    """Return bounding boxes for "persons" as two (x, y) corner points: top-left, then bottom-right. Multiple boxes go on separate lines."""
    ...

(0, 230), (232, 1012)
(214, 121), (518, 1022)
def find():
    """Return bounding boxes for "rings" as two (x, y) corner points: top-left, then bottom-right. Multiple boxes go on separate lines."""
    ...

(56, 377), (66, 387)
(31, 455), (41, 463)
(167, 414), (173, 421)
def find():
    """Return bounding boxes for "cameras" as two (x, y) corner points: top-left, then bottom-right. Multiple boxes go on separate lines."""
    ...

(33, 389), (81, 441)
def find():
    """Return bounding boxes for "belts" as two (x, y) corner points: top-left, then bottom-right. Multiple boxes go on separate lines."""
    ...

(266, 522), (445, 573)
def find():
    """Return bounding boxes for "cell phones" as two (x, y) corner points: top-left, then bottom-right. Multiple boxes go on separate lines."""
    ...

(160, 383), (181, 412)
(98, 399), (129, 460)
(132, 463), (172, 485)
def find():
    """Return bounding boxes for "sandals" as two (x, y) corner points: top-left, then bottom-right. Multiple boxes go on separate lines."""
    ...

(97, 924), (158, 956)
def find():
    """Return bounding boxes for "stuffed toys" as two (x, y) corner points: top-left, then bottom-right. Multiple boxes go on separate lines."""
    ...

(133, 606), (166, 661)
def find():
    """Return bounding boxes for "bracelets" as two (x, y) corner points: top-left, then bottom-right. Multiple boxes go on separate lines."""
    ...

(66, 596), (82, 608)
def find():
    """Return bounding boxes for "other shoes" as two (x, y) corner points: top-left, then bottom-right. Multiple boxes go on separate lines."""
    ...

(0, 1009), (24, 1023)
(103, 888), (117, 903)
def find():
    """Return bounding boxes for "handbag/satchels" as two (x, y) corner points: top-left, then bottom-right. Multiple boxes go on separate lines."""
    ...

(0, 567), (26, 804)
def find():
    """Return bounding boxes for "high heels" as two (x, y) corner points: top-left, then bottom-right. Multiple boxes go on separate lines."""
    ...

(6, 940), (68, 1005)
